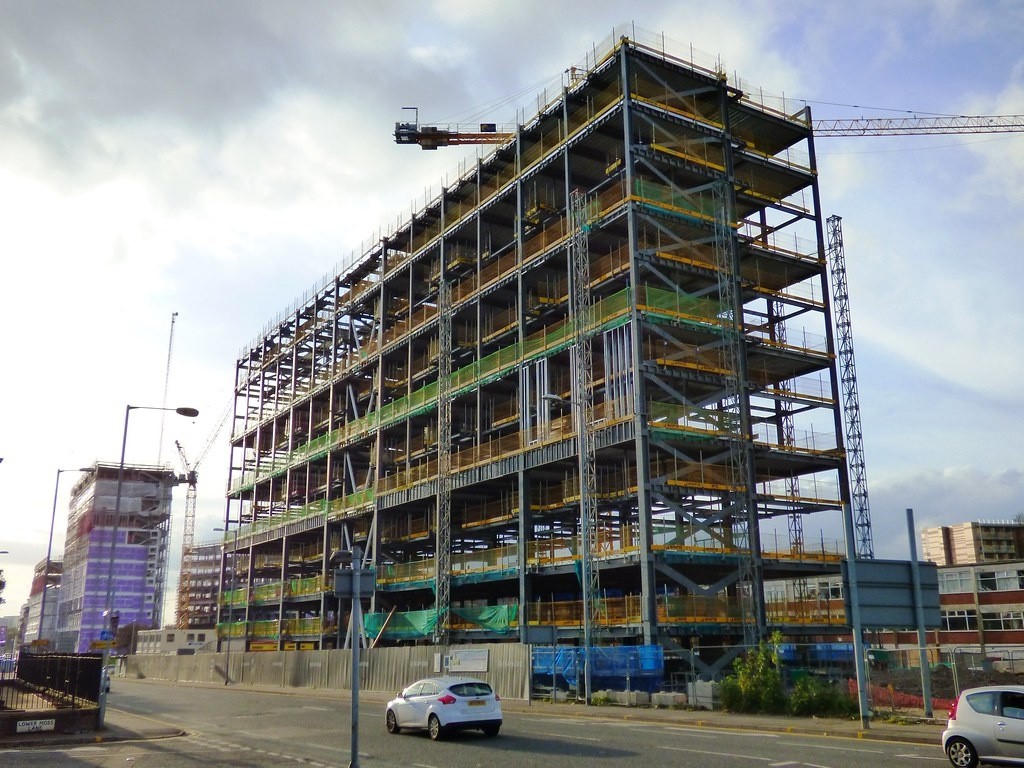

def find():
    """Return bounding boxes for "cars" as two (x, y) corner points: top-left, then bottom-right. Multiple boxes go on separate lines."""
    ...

(102, 668), (110, 692)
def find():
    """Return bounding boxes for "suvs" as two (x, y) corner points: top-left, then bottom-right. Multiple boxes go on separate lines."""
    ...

(942, 686), (1024, 768)
(385, 676), (503, 741)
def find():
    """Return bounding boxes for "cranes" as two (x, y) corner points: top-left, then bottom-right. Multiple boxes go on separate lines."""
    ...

(176, 397), (240, 630)
(396, 114), (1024, 599)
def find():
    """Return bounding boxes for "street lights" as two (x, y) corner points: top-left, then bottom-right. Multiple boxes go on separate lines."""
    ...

(101, 405), (199, 655)
(210, 527), (239, 686)
(36, 466), (95, 647)
